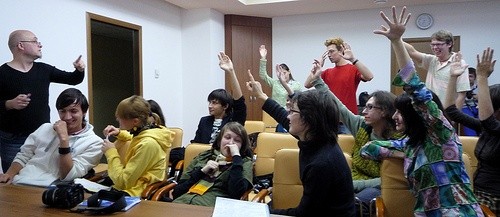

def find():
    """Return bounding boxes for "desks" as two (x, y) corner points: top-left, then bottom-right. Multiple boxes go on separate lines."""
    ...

(0, 184), (214, 217)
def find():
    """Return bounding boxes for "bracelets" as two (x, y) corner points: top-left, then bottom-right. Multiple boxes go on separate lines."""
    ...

(58, 146), (73, 154)
(287, 92), (296, 99)
(352, 59), (359, 65)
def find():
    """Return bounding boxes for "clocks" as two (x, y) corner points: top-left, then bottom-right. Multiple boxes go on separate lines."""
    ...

(416, 13), (433, 30)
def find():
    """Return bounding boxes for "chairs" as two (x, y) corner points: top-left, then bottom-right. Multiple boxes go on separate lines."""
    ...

(90, 111), (496, 217)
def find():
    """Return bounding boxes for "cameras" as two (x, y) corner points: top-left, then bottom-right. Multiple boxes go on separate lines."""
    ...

(41, 181), (85, 209)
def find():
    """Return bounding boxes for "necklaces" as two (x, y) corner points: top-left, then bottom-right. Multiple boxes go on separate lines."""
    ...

(440, 56), (451, 62)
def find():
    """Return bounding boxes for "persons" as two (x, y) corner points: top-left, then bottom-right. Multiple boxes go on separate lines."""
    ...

(257, 45), (304, 106)
(462, 67), (482, 136)
(0, 88), (104, 188)
(308, 57), (401, 215)
(443, 48), (499, 213)
(168, 122), (254, 207)
(358, 5), (485, 217)
(1, 29), (86, 175)
(304, 39), (371, 116)
(147, 99), (166, 127)
(101, 94), (174, 201)
(380, 25), (472, 132)
(192, 51), (248, 146)
(245, 68), (356, 217)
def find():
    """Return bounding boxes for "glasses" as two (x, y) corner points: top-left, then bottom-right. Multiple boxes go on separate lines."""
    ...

(431, 43), (446, 47)
(20, 40), (41, 45)
(363, 104), (382, 110)
(289, 108), (300, 115)
